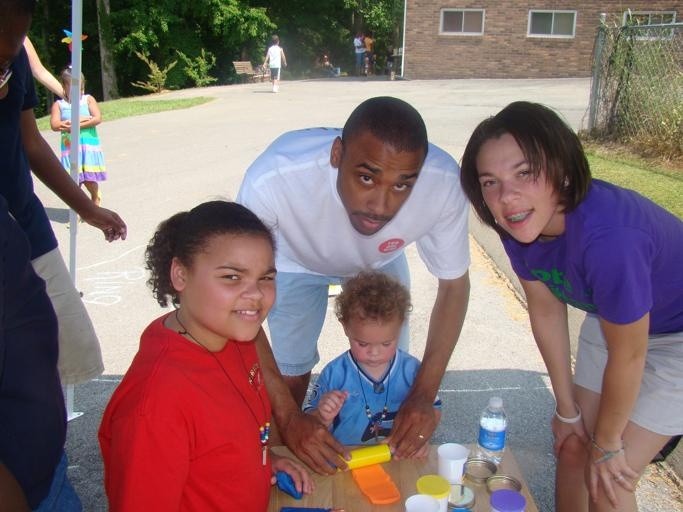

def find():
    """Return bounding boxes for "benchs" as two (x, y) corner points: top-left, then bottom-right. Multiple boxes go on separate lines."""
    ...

(232, 61), (262, 84)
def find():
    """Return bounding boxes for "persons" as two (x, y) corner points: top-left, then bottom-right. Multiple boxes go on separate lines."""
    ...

(21, 33), (66, 102)
(0, 2), (127, 511)
(353, 33), (366, 77)
(97, 201), (314, 511)
(302, 272), (441, 460)
(384, 46), (393, 75)
(51, 64), (107, 205)
(234, 96), (471, 478)
(262, 35), (287, 93)
(460, 101), (683, 511)
(363, 31), (373, 76)
(321, 56), (340, 77)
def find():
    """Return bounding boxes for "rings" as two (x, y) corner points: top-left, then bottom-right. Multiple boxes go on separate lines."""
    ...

(417, 434), (423, 439)
(614, 475), (623, 482)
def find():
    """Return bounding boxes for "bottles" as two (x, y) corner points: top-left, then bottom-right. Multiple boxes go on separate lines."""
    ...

(478, 396), (506, 462)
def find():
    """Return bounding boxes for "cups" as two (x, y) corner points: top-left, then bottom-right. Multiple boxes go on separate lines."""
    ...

(437, 443), (467, 481)
(416, 475), (448, 511)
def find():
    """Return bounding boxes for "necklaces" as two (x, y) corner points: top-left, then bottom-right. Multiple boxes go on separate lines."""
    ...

(354, 359), (391, 435)
(175, 309), (270, 466)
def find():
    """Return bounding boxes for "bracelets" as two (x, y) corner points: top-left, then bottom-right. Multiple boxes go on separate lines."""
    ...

(554, 402), (582, 423)
(590, 433), (625, 463)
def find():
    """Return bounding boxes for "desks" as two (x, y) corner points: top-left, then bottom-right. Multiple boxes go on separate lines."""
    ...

(266, 444), (540, 512)
